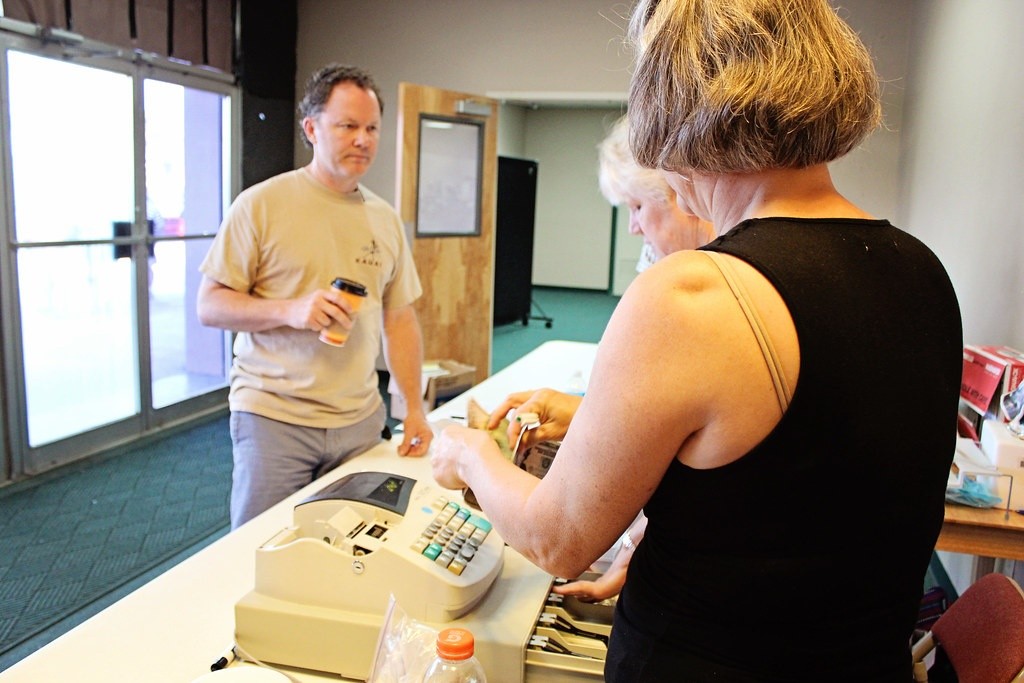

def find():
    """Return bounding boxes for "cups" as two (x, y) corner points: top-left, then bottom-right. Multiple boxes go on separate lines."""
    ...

(319, 276), (369, 348)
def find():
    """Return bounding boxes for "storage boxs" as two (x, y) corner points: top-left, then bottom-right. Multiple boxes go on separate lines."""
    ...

(389, 358), (477, 422)
(956, 345), (1024, 449)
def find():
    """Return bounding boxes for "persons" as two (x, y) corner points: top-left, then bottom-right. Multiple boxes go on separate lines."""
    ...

(430, 0), (964, 683)
(200, 62), (435, 536)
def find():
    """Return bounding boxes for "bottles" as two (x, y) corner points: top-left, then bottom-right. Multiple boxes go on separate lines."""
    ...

(421, 628), (487, 683)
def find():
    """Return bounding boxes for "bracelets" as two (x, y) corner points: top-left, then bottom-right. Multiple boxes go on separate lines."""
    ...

(621, 533), (636, 551)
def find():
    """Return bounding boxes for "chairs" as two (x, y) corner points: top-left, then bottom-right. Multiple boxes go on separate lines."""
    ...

(911, 573), (1024, 683)
